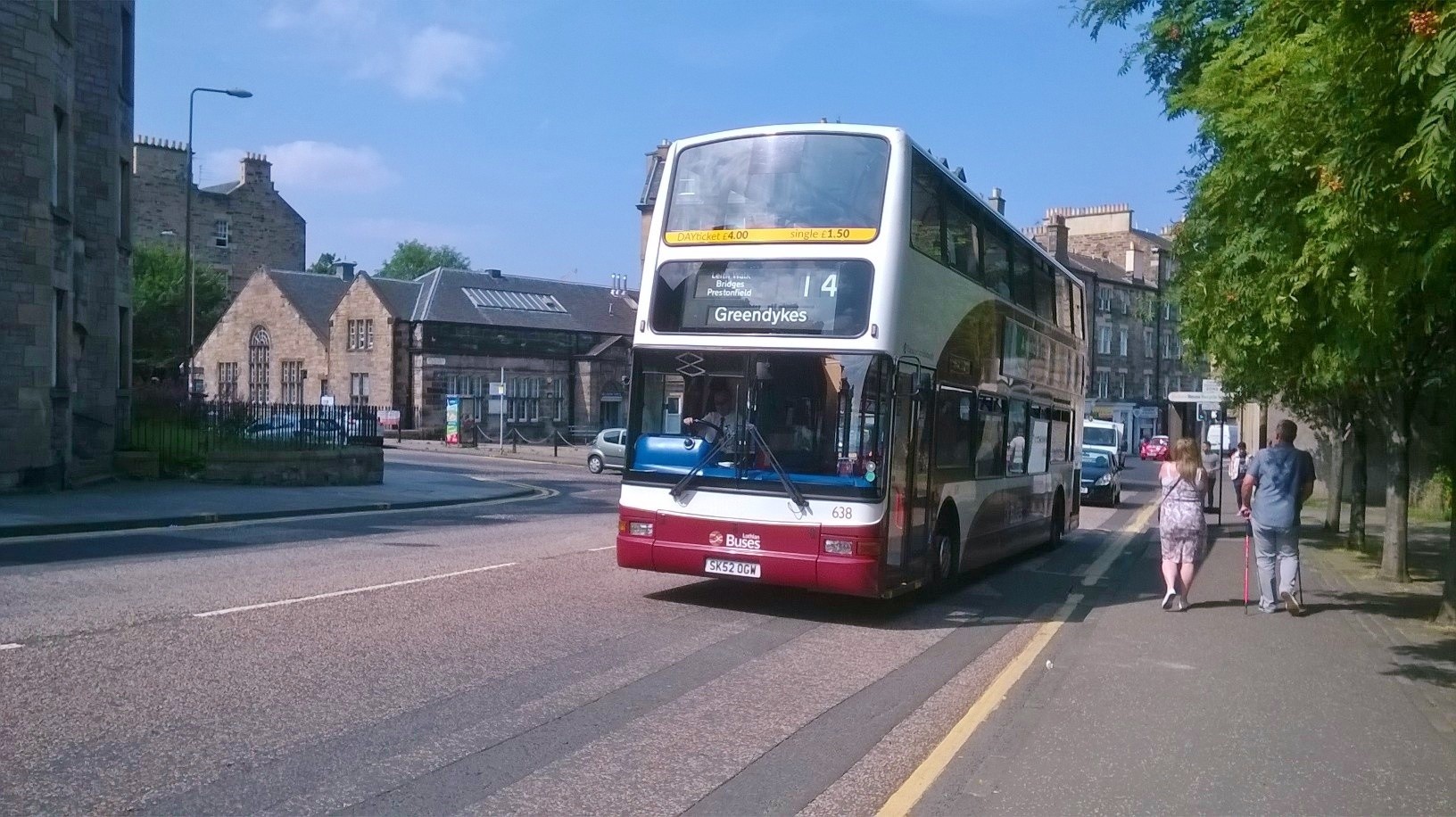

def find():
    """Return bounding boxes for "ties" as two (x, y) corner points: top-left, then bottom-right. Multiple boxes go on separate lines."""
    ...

(713, 417), (724, 447)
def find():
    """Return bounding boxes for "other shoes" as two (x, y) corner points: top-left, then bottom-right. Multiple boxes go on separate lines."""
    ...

(1162, 589), (1176, 609)
(1237, 511), (1242, 517)
(1257, 606), (1275, 613)
(1208, 502), (1213, 508)
(1281, 591), (1299, 617)
(1178, 602), (1190, 610)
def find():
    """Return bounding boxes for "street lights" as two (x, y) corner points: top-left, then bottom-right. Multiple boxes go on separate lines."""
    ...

(160, 230), (196, 392)
(179, 85), (254, 423)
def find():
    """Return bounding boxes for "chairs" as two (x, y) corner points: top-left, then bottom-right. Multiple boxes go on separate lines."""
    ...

(1096, 457), (1108, 465)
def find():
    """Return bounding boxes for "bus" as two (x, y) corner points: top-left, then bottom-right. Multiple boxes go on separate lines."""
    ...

(610, 113), (1091, 603)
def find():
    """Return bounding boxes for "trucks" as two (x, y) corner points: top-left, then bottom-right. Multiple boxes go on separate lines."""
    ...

(1082, 416), (1127, 472)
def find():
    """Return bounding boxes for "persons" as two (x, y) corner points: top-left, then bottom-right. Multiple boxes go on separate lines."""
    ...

(1239, 419), (1317, 616)
(1157, 435), (1210, 612)
(1200, 441), (1221, 509)
(1006, 427), (1027, 473)
(681, 388), (754, 470)
(1227, 440), (1255, 517)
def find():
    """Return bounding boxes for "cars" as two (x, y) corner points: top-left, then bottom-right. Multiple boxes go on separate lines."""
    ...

(585, 427), (628, 473)
(1139, 434), (1171, 462)
(236, 414), (346, 446)
(1080, 446), (1123, 508)
(337, 411), (384, 447)
